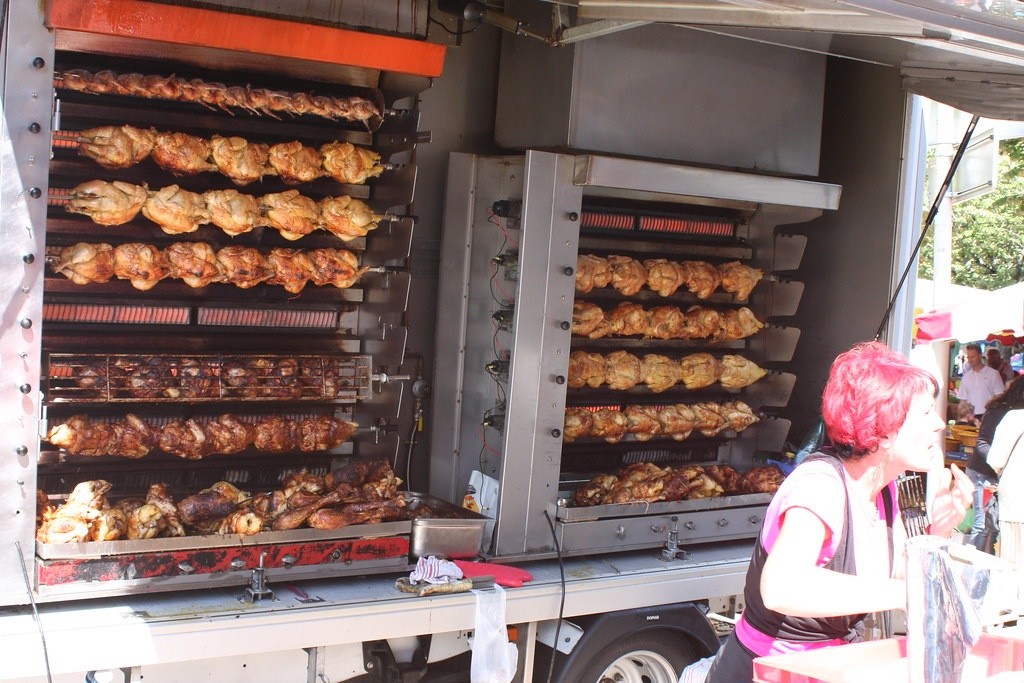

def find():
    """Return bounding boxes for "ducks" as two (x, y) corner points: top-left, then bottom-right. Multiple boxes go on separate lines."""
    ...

(36, 457), (433, 545)
(48, 412), (359, 460)
(576, 462), (786, 505)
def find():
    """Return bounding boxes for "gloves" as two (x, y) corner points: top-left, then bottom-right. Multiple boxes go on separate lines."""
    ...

(394, 576), (472, 597)
(453, 559), (533, 588)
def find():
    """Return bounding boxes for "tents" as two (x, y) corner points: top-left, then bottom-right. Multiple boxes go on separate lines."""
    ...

(913, 279), (1024, 345)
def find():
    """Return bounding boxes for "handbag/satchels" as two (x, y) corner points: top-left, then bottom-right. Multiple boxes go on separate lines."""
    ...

(985, 492), (1000, 532)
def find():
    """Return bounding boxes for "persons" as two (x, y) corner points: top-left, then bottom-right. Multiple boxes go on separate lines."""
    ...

(958, 343), (1024, 564)
(703, 342), (974, 683)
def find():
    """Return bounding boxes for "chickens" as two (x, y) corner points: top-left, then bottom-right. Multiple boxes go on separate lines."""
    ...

(66, 179), (149, 226)
(318, 195), (381, 242)
(201, 189), (269, 236)
(141, 183), (211, 235)
(64, 69), (384, 134)
(150, 130), (218, 177)
(50, 241), (370, 291)
(572, 299), (765, 341)
(268, 141), (331, 185)
(76, 356), (340, 398)
(79, 124), (158, 170)
(210, 134), (278, 185)
(574, 254), (764, 300)
(562, 400), (760, 443)
(318, 140), (384, 185)
(258, 189), (325, 240)
(567, 350), (767, 393)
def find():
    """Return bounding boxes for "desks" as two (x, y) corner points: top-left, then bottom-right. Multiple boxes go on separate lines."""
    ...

(753, 638), (1023, 683)
(945, 451), (972, 468)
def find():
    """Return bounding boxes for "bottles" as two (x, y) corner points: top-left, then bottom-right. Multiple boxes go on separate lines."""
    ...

(794, 415), (824, 470)
